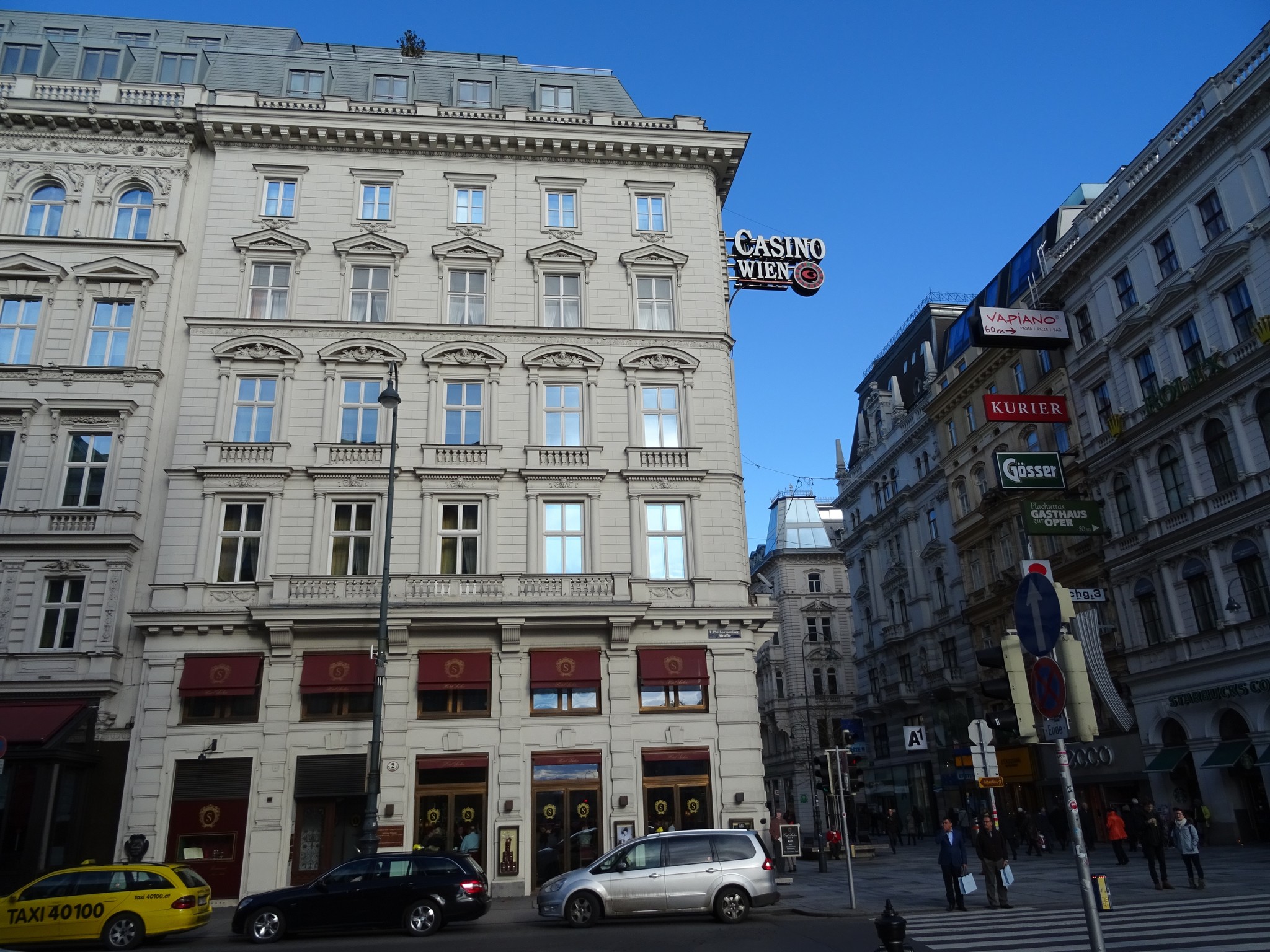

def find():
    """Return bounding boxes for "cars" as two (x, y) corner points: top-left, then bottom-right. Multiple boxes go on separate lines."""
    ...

(231, 849), (493, 945)
(534, 828), (600, 874)
(536, 828), (781, 928)
(0, 859), (213, 952)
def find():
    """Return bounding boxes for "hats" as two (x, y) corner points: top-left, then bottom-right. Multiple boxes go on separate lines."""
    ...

(776, 809), (781, 813)
(1018, 808), (1022, 811)
(831, 825), (836, 832)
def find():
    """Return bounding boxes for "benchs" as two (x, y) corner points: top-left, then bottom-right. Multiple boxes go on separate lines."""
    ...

(800, 833), (890, 859)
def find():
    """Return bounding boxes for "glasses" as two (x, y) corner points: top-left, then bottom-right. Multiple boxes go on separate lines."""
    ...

(1175, 813), (1183, 816)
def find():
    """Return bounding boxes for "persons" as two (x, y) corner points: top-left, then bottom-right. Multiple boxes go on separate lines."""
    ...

(1137, 801), (1175, 890)
(783, 812), (796, 872)
(946, 800), (1217, 875)
(1173, 809), (1205, 890)
(827, 824), (842, 860)
(935, 815), (969, 911)
(976, 816), (1015, 909)
(536, 820), (597, 883)
(770, 808), (800, 875)
(424, 823), (482, 853)
(884, 804), (924, 854)
(648, 817), (675, 833)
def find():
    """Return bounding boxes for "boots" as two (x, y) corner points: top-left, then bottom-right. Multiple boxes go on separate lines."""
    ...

(1188, 878), (1197, 888)
(1013, 853), (1017, 860)
(1196, 878), (1205, 889)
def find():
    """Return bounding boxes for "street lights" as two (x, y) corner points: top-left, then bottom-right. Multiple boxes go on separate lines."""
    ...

(801, 632), (838, 873)
(789, 724), (819, 848)
(927, 676), (975, 849)
(1225, 577), (1270, 629)
(361, 360), (404, 856)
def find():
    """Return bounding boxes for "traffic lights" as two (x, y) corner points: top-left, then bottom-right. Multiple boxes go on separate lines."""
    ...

(813, 752), (834, 796)
(845, 751), (865, 795)
(842, 730), (855, 747)
(975, 634), (1039, 745)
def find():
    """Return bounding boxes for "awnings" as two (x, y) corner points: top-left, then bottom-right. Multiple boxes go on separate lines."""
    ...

(1141, 746), (1188, 773)
(1199, 738), (1252, 769)
(1253, 745), (1270, 767)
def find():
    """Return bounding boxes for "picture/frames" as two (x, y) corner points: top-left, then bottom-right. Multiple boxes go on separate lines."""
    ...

(780, 824), (802, 857)
(614, 820), (635, 846)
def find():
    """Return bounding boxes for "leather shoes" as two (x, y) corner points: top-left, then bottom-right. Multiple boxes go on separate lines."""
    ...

(947, 904), (955, 911)
(958, 905), (967, 911)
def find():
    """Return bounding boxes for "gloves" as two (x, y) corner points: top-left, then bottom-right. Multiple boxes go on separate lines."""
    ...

(778, 837), (782, 842)
(796, 823), (800, 827)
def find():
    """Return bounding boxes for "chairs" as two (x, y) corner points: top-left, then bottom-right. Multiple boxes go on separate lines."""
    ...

(468, 848), (481, 864)
(579, 844), (596, 868)
(363, 863), (380, 882)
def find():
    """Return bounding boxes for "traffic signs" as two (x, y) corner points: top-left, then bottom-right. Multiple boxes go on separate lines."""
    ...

(978, 777), (1004, 788)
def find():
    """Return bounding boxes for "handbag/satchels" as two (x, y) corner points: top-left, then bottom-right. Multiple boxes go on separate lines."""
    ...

(1037, 834), (1045, 849)
(826, 842), (830, 847)
(958, 867), (977, 894)
(1000, 864), (1014, 886)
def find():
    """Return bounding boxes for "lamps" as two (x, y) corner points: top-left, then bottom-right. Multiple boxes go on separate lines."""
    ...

(738, 823), (746, 829)
(384, 804), (394, 818)
(197, 737), (217, 761)
(505, 799), (513, 811)
(736, 792), (745, 802)
(620, 795), (627, 805)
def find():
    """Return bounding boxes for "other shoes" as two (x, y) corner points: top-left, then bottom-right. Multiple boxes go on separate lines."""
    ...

(1162, 880), (1176, 889)
(1025, 842), (1096, 856)
(1116, 861), (1124, 865)
(1155, 883), (1163, 890)
(788, 869), (792, 872)
(893, 852), (896, 854)
(1001, 902), (1014, 908)
(891, 836), (924, 846)
(793, 868), (797, 872)
(1122, 858), (1130, 865)
(991, 904), (997, 909)
